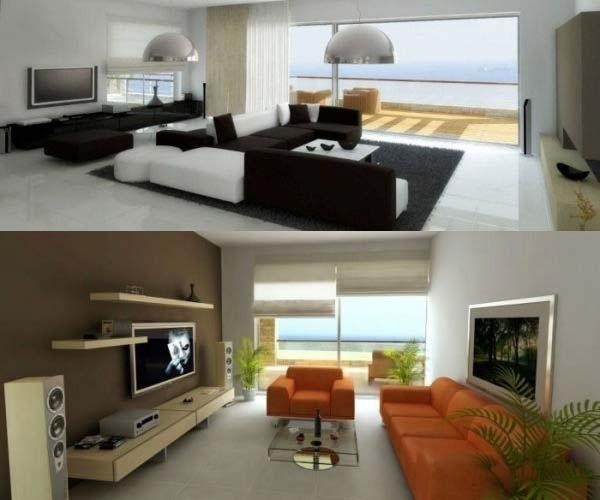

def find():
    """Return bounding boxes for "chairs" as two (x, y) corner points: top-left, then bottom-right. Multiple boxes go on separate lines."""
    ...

(367, 350), (394, 385)
(263, 364), (355, 421)
(288, 85), (381, 116)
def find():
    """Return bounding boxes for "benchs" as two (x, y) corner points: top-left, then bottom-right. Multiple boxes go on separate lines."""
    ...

(42, 126), (134, 167)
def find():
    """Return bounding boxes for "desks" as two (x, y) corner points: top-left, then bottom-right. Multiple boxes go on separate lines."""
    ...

(538, 131), (599, 230)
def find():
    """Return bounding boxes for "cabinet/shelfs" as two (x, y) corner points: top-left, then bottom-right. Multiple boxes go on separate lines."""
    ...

(67, 384), (248, 482)
(2, 110), (113, 153)
(51, 290), (213, 351)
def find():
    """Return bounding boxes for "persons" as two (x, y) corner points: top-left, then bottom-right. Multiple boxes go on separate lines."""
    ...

(159, 326), (192, 382)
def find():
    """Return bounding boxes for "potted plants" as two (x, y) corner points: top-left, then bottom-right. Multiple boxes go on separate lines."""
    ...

(231, 335), (269, 402)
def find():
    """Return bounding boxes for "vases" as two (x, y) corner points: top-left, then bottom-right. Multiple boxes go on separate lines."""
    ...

(184, 283), (200, 303)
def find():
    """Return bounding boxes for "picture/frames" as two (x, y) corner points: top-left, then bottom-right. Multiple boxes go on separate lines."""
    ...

(462, 293), (559, 413)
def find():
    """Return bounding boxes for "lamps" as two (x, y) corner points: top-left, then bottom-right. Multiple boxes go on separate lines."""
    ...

(142, 1), (201, 66)
(323, 0), (395, 67)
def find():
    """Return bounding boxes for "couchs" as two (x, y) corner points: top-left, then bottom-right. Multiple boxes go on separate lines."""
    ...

(114, 99), (409, 220)
(378, 377), (590, 500)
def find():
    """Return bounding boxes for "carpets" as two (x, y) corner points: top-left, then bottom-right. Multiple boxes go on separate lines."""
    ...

(87, 136), (467, 232)
